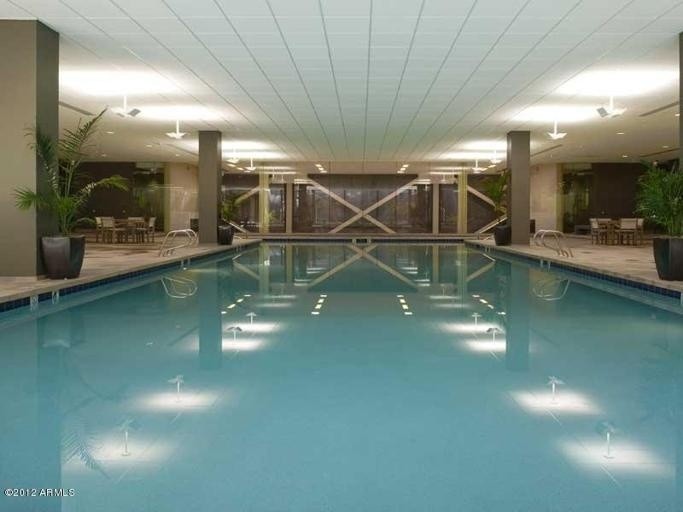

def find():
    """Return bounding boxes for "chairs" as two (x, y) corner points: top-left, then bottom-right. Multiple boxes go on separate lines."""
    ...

(589, 215), (645, 246)
(93, 216), (156, 244)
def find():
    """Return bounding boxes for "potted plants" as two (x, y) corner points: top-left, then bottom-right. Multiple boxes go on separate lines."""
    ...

(632, 159), (682, 283)
(217, 191), (249, 244)
(10, 107), (130, 279)
(482, 169), (510, 246)
